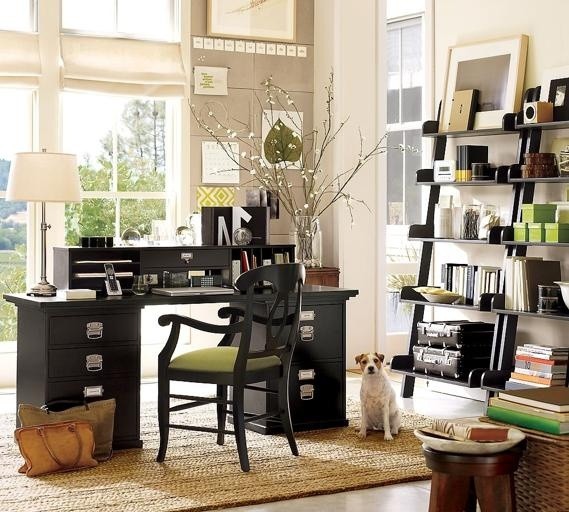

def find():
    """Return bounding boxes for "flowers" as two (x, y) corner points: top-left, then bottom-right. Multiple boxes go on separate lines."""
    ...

(186, 68), (421, 266)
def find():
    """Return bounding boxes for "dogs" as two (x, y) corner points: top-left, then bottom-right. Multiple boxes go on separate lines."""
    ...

(354, 352), (402, 441)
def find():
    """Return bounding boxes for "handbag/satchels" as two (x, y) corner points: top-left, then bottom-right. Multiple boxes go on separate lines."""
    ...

(15, 398), (116, 477)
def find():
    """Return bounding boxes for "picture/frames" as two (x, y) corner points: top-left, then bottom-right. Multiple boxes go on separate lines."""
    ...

(206, 0), (296, 43)
(438, 34), (528, 133)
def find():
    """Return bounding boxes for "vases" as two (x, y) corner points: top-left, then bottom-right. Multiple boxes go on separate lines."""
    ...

(294, 215), (322, 267)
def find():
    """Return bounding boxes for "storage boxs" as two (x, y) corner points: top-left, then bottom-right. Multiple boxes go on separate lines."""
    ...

(513, 204), (569, 243)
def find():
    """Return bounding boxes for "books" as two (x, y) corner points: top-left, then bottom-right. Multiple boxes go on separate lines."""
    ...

(56, 289), (96, 299)
(418, 256), (569, 446)
(224, 251), (289, 285)
(456, 145), (489, 182)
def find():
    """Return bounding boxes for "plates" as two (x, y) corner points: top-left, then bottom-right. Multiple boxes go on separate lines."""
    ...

(421, 293), (463, 303)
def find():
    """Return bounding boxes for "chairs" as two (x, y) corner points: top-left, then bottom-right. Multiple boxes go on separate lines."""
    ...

(156, 262), (305, 471)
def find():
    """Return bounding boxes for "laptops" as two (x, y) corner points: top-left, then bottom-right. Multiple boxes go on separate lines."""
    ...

(151, 286), (235, 297)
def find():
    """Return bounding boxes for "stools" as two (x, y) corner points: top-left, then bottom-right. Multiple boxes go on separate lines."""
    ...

(421, 442), (522, 512)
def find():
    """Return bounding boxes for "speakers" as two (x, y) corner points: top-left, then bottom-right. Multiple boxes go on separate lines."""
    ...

(523, 101), (553, 124)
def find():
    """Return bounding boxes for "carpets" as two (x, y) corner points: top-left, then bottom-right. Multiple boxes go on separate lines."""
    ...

(0, 392), (444, 512)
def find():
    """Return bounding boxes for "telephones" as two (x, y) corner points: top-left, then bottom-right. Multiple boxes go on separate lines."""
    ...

(104, 263), (122, 296)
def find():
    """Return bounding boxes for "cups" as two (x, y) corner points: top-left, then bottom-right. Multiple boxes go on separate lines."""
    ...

(471, 163), (490, 178)
(537, 284), (561, 315)
(434, 202), (500, 239)
(82, 236), (113, 247)
(132, 275), (153, 295)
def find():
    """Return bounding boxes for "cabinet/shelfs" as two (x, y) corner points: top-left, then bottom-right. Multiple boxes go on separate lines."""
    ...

(389, 119), (569, 414)
(52, 244), (296, 289)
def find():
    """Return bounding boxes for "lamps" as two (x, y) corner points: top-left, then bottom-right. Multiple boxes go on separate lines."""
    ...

(4, 149), (81, 297)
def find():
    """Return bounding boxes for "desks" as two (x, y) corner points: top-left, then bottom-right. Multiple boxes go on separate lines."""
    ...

(4, 283), (357, 452)
(305, 266), (340, 286)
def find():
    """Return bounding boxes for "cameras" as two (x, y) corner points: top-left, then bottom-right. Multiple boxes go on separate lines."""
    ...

(143, 274), (158, 285)
